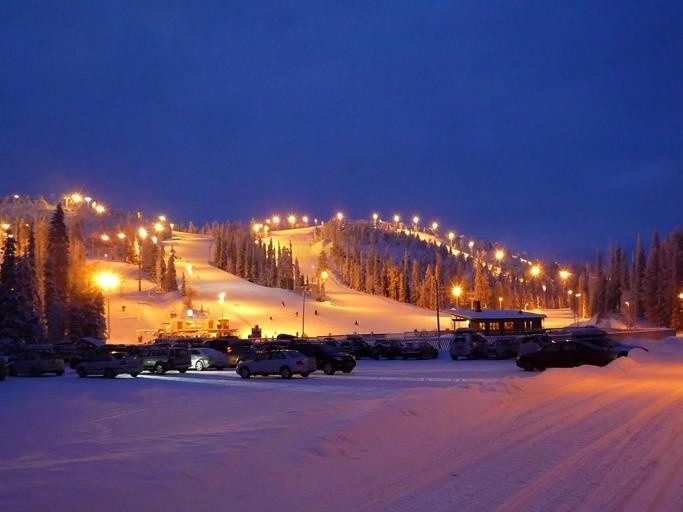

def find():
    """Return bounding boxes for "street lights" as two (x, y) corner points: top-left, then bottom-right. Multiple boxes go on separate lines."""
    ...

(71, 193), (192, 340)
(559, 270), (581, 326)
(624, 302), (629, 328)
(252, 216), (317, 245)
(320, 271), (327, 297)
(372, 213), (539, 318)
(218, 293), (224, 329)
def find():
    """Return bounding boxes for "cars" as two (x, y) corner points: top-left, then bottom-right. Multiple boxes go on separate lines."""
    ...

(449, 325), (627, 372)
(226, 337), (437, 379)
(9, 351), (65, 377)
(76, 350), (143, 379)
(187, 347), (226, 371)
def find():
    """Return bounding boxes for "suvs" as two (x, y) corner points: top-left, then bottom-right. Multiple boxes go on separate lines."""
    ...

(138, 346), (191, 375)
